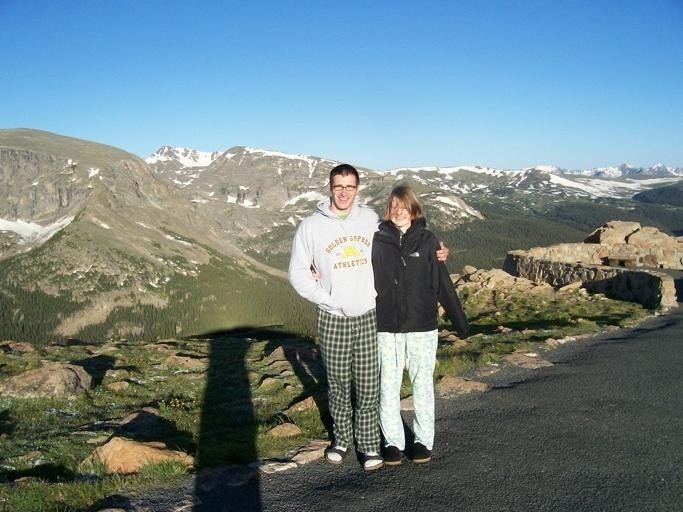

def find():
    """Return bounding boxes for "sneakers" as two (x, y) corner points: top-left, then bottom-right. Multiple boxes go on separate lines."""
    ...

(410, 441), (432, 463)
(382, 444), (403, 466)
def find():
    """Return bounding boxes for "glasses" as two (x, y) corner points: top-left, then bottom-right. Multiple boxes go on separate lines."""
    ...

(331, 184), (358, 192)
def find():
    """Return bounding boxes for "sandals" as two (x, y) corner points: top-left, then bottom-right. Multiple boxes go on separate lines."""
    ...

(362, 448), (383, 471)
(324, 441), (350, 466)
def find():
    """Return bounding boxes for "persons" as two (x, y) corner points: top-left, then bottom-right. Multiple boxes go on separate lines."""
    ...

(287, 163), (449, 470)
(370, 186), (471, 466)
